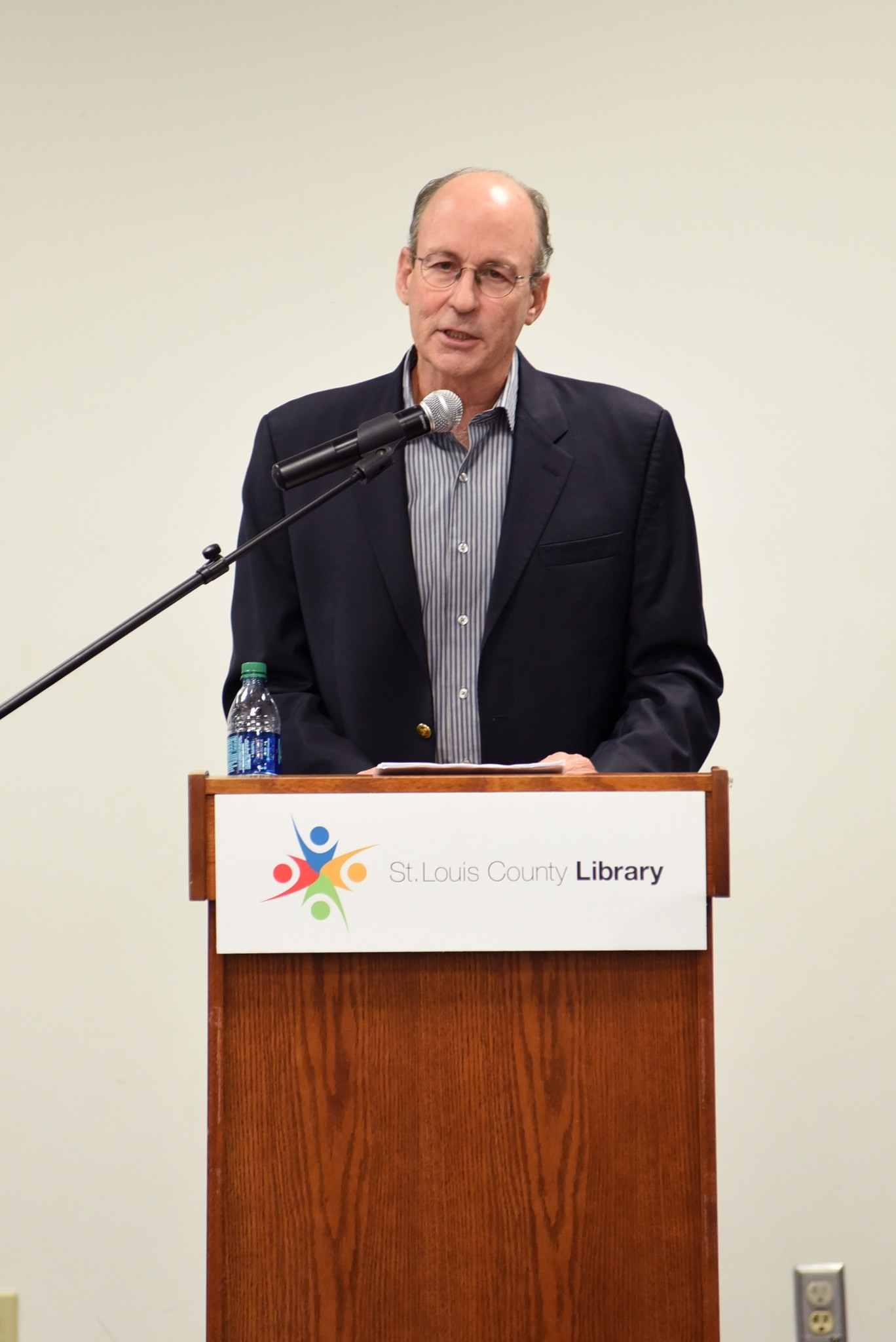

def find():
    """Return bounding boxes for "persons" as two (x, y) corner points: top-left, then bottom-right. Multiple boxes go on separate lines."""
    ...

(223, 168), (726, 774)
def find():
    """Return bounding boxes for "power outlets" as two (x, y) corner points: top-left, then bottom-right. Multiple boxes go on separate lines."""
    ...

(795, 1261), (848, 1342)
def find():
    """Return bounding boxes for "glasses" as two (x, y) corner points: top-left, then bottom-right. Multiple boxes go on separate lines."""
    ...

(409, 251), (539, 299)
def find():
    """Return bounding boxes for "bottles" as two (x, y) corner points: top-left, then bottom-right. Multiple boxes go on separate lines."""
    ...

(226, 662), (283, 778)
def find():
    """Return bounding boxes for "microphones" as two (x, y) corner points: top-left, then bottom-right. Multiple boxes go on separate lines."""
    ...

(270, 388), (463, 494)
